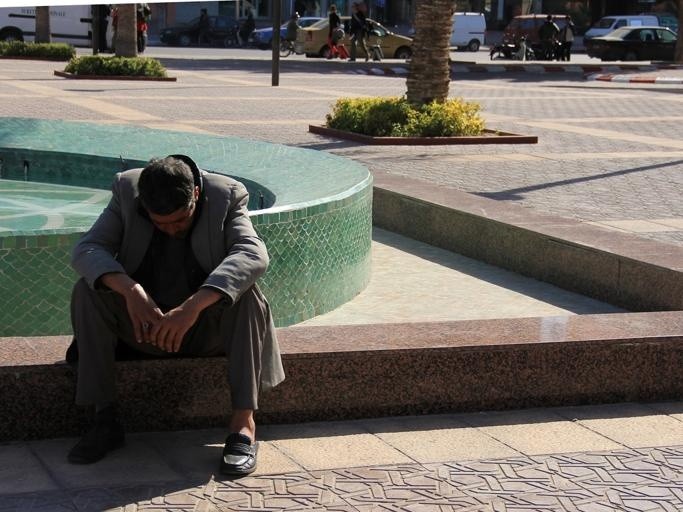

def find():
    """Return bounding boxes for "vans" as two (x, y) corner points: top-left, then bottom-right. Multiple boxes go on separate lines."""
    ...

(501, 13), (572, 58)
(1, 4), (151, 53)
(408, 11), (486, 53)
(582, 14), (659, 48)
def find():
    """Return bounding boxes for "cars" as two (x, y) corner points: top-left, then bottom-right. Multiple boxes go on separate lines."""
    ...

(251, 16), (325, 50)
(293, 15), (413, 59)
(159, 15), (256, 47)
(583, 25), (678, 62)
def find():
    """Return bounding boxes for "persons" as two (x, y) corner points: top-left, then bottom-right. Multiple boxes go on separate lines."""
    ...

(558, 15), (577, 60)
(238, 11), (256, 47)
(287, 12), (303, 41)
(538, 13), (560, 60)
(347, 2), (371, 62)
(91, 3), (152, 55)
(199, 7), (209, 26)
(326, 5), (342, 58)
(64, 153), (286, 478)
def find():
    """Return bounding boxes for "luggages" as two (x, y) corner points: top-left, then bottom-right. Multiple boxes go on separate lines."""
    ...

(336, 44), (349, 59)
(367, 41), (384, 61)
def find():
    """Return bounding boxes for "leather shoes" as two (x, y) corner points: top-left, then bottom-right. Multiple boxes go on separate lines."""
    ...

(221, 432), (258, 476)
(68, 427), (125, 465)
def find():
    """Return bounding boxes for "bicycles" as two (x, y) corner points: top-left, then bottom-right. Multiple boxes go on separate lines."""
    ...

(278, 35), (296, 58)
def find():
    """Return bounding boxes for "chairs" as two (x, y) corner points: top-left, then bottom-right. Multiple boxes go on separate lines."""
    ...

(644, 33), (653, 39)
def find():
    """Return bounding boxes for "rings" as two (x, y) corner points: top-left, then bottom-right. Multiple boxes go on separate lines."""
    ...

(143, 323), (151, 328)
